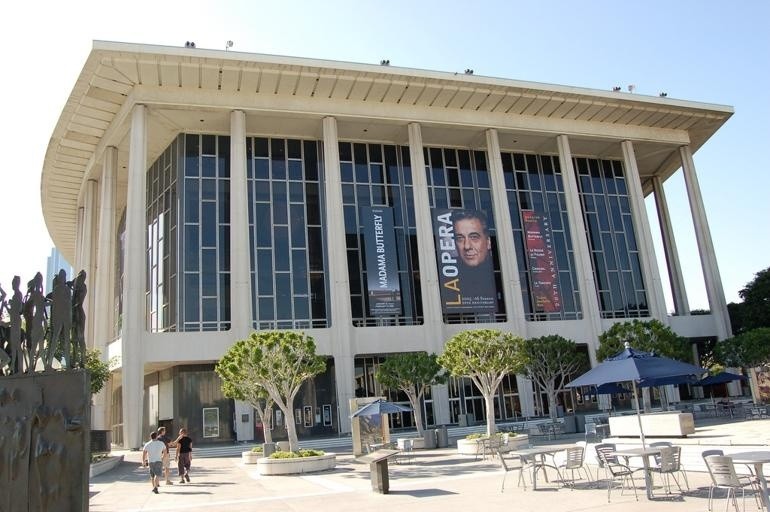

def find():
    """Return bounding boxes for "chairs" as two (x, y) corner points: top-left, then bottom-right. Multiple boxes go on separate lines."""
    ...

(364, 435), (414, 464)
(594, 443), (770, 512)
(478, 421), (592, 495)
(665, 398), (769, 423)
(582, 411), (619, 439)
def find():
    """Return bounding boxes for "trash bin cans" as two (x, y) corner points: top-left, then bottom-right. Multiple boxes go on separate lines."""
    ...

(564, 416), (577, 433)
(557, 406), (564, 418)
(424, 430), (436, 449)
(458, 415), (466, 426)
(575, 416), (586, 433)
(436, 428), (448, 448)
(466, 414), (473, 425)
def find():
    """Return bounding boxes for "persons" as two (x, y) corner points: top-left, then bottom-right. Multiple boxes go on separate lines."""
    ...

(143, 432), (167, 493)
(439, 209), (499, 312)
(0, 268), (87, 375)
(157, 427), (183, 485)
(175, 428), (192, 483)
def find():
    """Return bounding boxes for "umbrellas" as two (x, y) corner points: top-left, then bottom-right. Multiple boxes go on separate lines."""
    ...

(580, 384), (635, 416)
(351, 398), (415, 444)
(564, 342), (710, 478)
(692, 371), (752, 402)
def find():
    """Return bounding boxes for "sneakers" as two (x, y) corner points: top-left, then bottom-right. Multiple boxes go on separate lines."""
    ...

(185, 474), (190, 482)
(166, 480), (173, 485)
(179, 480), (185, 483)
(152, 487), (158, 494)
